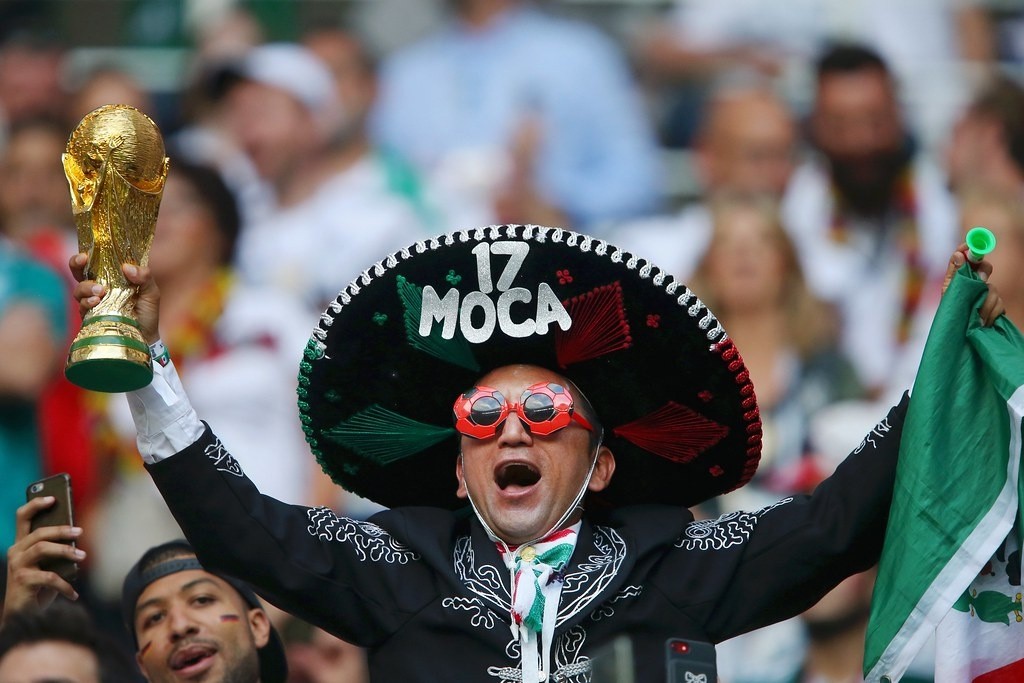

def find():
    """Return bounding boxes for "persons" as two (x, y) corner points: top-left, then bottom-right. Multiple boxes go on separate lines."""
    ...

(70, 222), (1007, 682)
(0, 0), (1024, 683)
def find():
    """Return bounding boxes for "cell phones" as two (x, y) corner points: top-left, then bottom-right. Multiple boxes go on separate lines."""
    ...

(26, 472), (78, 584)
(666, 638), (718, 683)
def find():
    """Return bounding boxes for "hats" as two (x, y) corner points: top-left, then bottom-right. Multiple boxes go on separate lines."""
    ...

(123, 539), (289, 683)
(248, 44), (340, 146)
(296, 225), (763, 510)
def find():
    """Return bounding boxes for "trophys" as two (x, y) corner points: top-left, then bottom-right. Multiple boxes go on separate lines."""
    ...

(62, 102), (170, 393)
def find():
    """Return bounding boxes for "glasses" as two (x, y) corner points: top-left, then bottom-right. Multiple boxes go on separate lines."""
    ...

(449, 381), (595, 440)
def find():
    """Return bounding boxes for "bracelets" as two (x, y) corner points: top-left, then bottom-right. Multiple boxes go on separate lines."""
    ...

(148, 337), (164, 360)
(154, 347), (170, 368)
